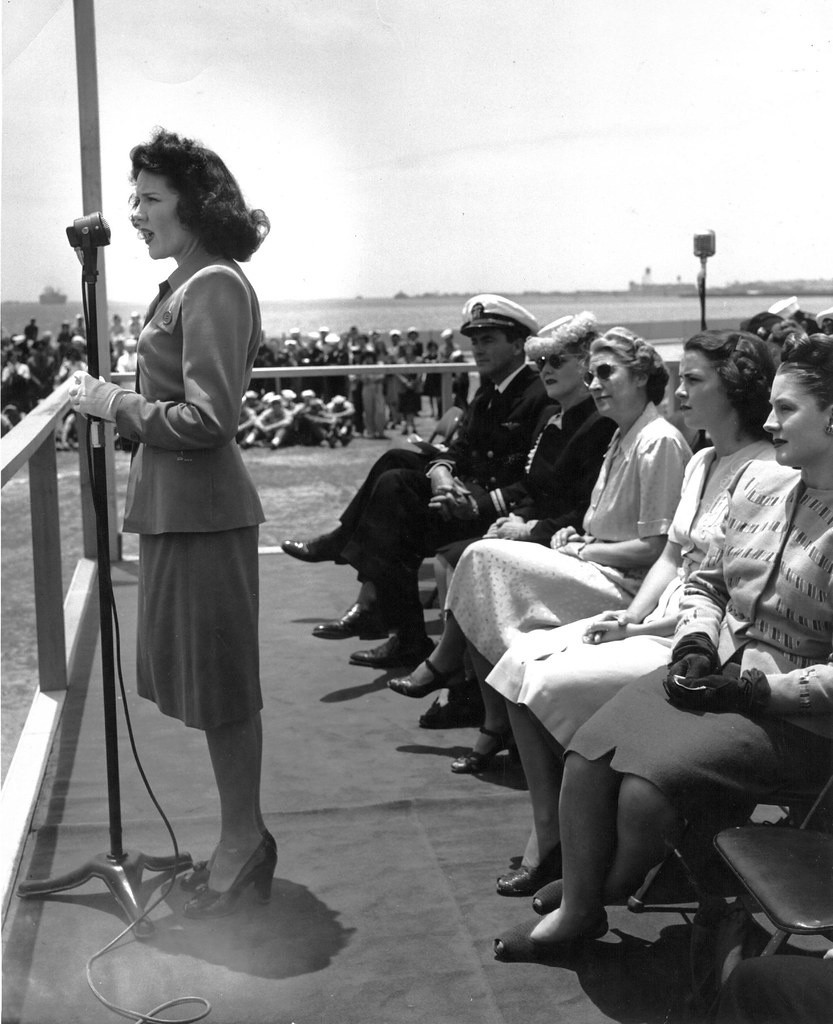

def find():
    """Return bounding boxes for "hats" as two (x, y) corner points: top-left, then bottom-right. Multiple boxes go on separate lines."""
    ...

(459, 293), (538, 337)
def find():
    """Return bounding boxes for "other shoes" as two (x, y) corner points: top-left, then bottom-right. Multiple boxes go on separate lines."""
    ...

(494, 864), (562, 894)
(419, 685), (482, 728)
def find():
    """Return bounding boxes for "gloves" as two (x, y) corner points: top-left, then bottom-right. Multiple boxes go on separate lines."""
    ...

(667, 633), (721, 696)
(662, 668), (772, 719)
(69, 370), (137, 423)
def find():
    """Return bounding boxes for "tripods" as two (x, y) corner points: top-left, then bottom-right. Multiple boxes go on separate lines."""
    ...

(15, 248), (196, 939)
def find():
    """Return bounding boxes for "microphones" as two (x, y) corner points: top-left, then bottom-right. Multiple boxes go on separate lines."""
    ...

(65, 211), (111, 249)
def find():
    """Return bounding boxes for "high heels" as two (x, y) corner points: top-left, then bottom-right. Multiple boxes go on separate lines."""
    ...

(450, 724), (523, 774)
(175, 828), (277, 890)
(182, 837), (276, 919)
(386, 658), (466, 703)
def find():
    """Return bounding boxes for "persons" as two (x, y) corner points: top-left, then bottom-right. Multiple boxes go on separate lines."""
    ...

(281, 290), (833, 1024)
(1, 312), (470, 447)
(68, 129), (278, 921)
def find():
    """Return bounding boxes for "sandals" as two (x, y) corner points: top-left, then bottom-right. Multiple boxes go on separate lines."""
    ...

(532, 878), (565, 915)
(494, 908), (610, 960)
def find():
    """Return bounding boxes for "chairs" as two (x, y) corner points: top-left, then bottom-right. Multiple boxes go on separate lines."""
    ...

(421, 409), (833, 1024)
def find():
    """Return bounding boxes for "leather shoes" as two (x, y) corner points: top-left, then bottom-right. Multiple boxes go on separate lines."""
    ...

(348, 636), (435, 667)
(281, 534), (349, 565)
(312, 603), (389, 640)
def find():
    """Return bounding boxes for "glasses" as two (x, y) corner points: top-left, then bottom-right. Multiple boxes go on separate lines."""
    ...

(535, 354), (578, 369)
(581, 363), (633, 387)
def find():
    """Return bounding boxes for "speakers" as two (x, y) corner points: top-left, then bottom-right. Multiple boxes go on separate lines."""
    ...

(693, 230), (717, 257)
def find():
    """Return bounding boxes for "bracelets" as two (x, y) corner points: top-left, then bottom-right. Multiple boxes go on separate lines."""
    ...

(578, 543), (588, 561)
